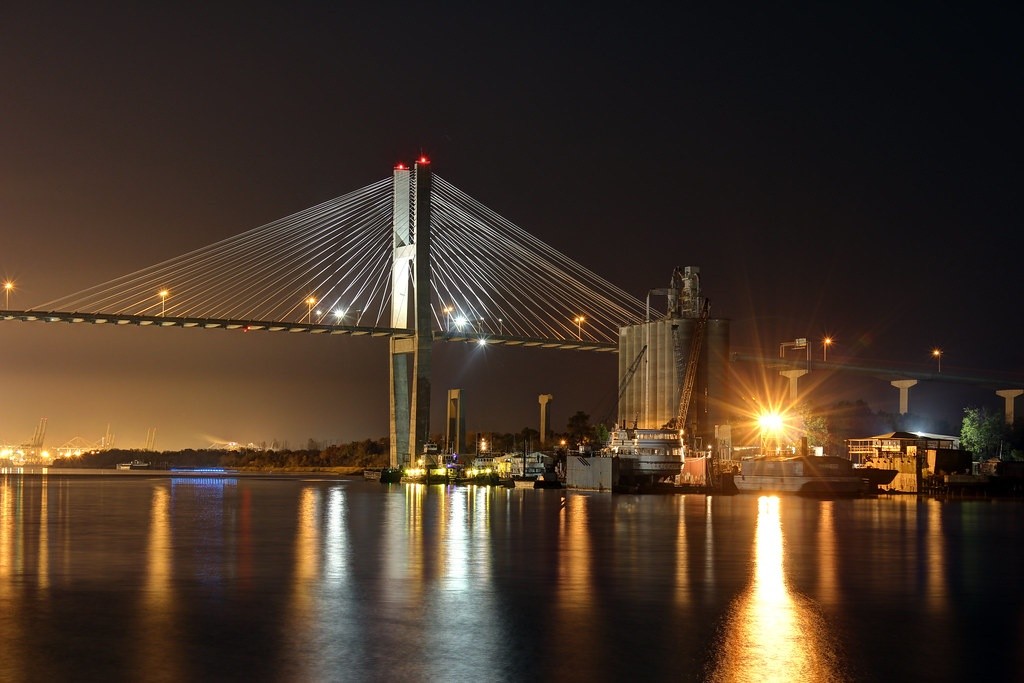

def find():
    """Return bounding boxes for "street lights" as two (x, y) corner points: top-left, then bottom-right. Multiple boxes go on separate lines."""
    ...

(574, 317), (584, 341)
(456, 317), (465, 332)
(307, 297), (314, 323)
(823, 339), (830, 361)
(5, 283), (12, 310)
(498, 319), (502, 335)
(444, 308), (453, 331)
(335, 310), (343, 325)
(934, 350), (940, 374)
(160, 290), (167, 317)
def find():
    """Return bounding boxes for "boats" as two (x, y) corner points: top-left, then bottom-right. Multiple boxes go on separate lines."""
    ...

(379, 467), (405, 484)
(732, 453), (899, 496)
